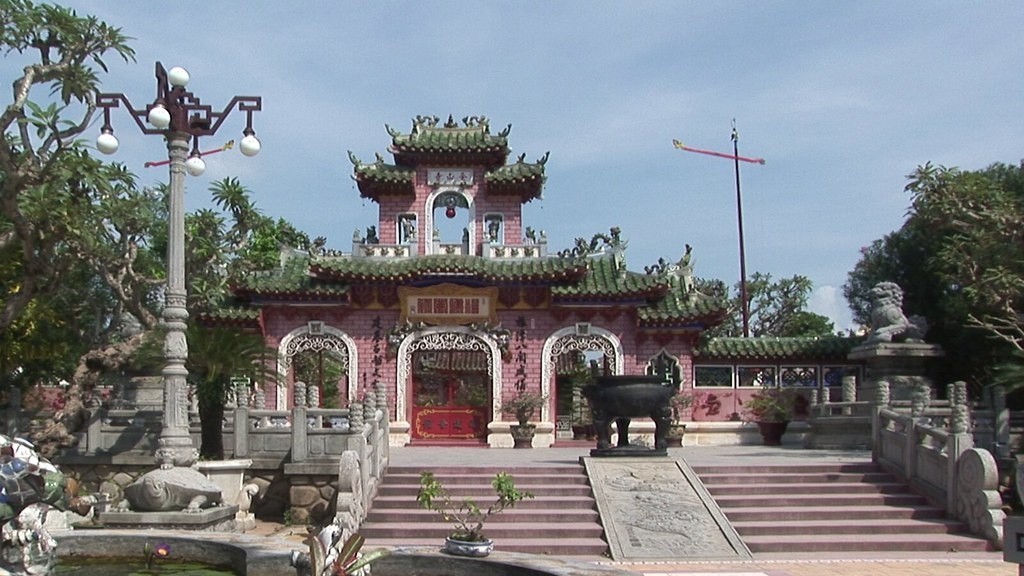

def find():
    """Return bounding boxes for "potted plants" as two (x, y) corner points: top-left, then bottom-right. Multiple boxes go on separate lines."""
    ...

(182, 328), (278, 501)
(662, 392), (694, 448)
(569, 403), (597, 440)
(495, 393), (546, 449)
(744, 385), (799, 449)
(412, 470), (533, 557)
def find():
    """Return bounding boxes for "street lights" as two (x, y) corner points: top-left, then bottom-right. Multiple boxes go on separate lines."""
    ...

(96, 60), (262, 468)
